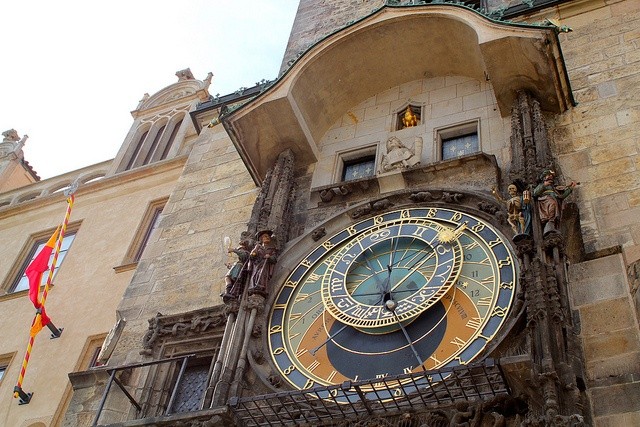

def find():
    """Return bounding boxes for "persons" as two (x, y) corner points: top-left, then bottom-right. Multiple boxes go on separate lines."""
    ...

(491, 181), (530, 240)
(245, 228), (278, 299)
(218, 229), (254, 302)
(376, 134), (424, 172)
(533, 168), (580, 235)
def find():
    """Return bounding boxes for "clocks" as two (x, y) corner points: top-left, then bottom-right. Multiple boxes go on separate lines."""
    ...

(267, 204), (522, 405)
